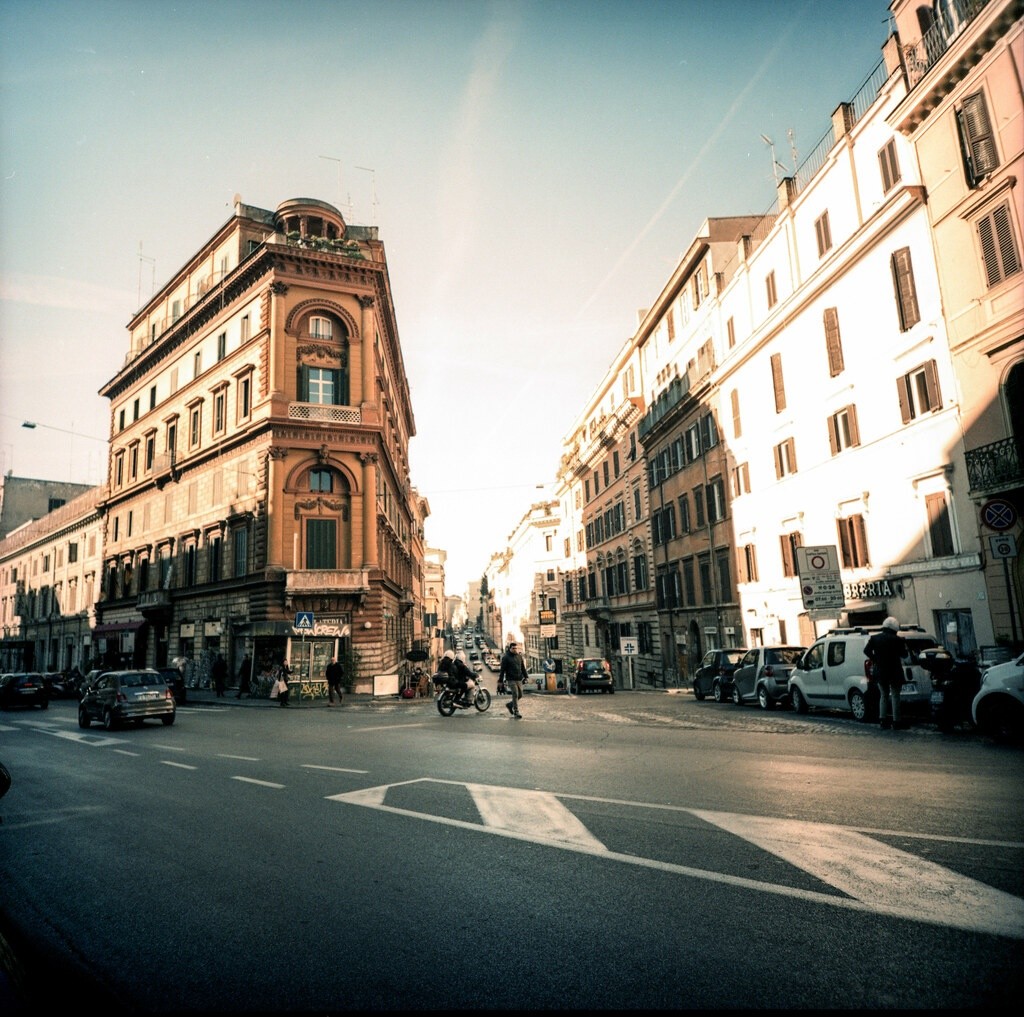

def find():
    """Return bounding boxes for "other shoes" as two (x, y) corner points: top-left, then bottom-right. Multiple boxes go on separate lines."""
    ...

(879, 719), (891, 729)
(328, 702), (336, 706)
(280, 703), (286, 706)
(513, 710), (522, 719)
(285, 702), (290, 705)
(891, 721), (911, 730)
(506, 703), (514, 716)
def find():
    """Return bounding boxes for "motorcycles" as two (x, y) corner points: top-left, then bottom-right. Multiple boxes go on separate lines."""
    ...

(432, 671), (492, 717)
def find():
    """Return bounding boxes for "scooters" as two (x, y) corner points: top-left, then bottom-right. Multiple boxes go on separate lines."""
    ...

(918, 647), (986, 730)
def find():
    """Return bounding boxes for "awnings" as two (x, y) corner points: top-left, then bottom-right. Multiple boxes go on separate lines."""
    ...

(92, 621), (145, 637)
(798, 601), (883, 617)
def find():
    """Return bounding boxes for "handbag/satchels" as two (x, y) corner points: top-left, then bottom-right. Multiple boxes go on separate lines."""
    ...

(209, 679), (216, 689)
(278, 680), (288, 694)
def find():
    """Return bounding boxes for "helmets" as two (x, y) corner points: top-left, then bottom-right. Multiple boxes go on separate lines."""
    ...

(443, 650), (455, 661)
(455, 650), (465, 663)
(881, 616), (900, 632)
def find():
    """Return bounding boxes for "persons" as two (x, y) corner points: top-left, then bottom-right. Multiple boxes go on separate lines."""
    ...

(415, 667), (425, 698)
(439, 650), (479, 707)
(211, 654), (228, 697)
(326, 658), (344, 705)
(235, 654), (251, 700)
(278, 660), (294, 706)
(863, 617), (911, 730)
(499, 643), (529, 718)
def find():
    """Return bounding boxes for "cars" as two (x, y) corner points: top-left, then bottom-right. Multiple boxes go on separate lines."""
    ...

(691, 648), (752, 704)
(143, 668), (187, 708)
(729, 645), (809, 711)
(452, 626), (501, 674)
(42, 669), (109, 701)
(76, 669), (176, 732)
(0, 672), (50, 710)
(969, 652), (1023, 741)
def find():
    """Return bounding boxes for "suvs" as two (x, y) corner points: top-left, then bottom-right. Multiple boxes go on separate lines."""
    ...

(786, 624), (936, 723)
(571, 658), (615, 695)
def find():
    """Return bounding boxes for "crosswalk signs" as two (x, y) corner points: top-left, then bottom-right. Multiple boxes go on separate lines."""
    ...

(295, 612), (315, 629)
